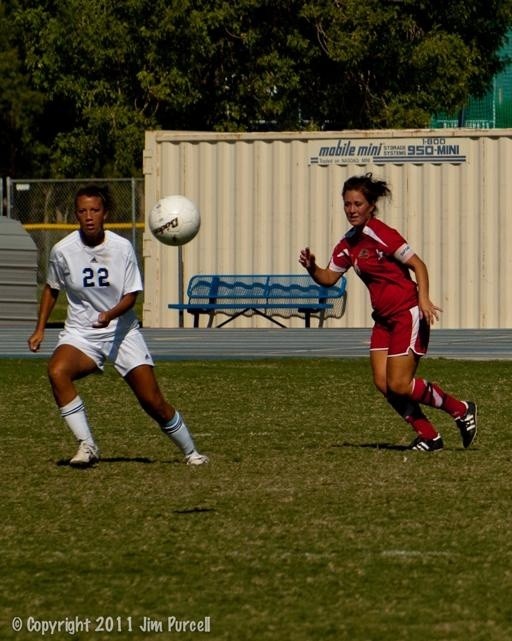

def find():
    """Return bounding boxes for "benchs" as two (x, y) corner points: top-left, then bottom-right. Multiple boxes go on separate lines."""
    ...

(168, 274), (347, 328)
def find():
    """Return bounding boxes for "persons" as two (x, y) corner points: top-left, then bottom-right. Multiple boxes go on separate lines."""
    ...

(26, 182), (210, 470)
(298, 171), (479, 455)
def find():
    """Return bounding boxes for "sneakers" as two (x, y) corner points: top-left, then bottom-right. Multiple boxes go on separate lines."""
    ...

(408, 432), (444, 451)
(454, 400), (478, 448)
(184, 451), (210, 465)
(70, 441), (98, 464)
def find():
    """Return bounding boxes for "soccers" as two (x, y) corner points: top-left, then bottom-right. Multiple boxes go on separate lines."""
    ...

(149, 194), (200, 245)
